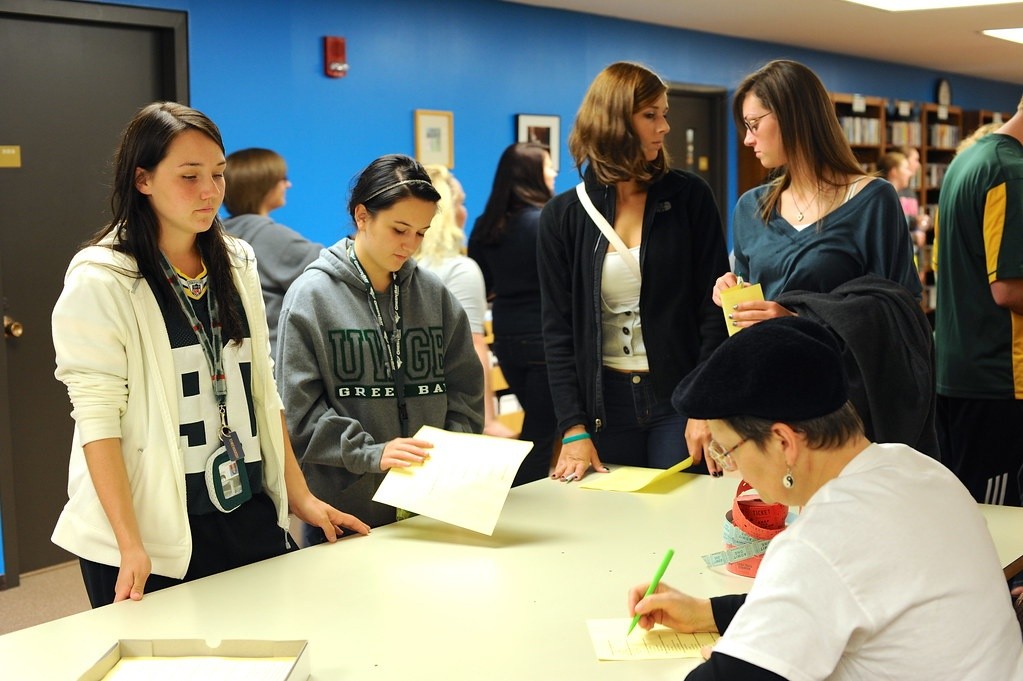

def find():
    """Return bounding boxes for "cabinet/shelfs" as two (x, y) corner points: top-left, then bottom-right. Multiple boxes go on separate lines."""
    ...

(736, 85), (1014, 322)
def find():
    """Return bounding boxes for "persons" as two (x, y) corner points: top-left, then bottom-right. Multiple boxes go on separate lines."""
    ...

(51, 102), (370, 609)
(467, 142), (558, 486)
(712, 60), (924, 328)
(221, 147), (326, 375)
(411, 164), (514, 436)
(628, 316), (1023, 681)
(933, 96), (1023, 507)
(275, 153), (485, 548)
(878, 145), (930, 281)
(537, 62), (731, 483)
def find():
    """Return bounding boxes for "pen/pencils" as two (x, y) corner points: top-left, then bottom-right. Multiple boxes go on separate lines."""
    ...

(736, 271), (743, 286)
(627, 547), (675, 636)
(565, 472), (575, 483)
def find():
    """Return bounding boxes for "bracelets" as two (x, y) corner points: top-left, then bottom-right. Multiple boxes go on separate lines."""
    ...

(574, 425), (585, 428)
(562, 432), (591, 444)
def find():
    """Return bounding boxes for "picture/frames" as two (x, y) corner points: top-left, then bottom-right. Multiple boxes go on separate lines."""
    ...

(415, 104), (455, 168)
(518, 114), (559, 176)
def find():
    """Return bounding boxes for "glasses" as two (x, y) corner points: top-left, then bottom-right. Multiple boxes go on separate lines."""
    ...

(707, 436), (746, 474)
(743, 111), (773, 132)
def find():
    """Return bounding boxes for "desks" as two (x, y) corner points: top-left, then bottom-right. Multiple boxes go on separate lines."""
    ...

(1, 467), (1022, 681)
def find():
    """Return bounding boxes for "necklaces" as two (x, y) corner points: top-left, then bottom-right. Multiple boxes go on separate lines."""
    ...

(791, 183), (827, 221)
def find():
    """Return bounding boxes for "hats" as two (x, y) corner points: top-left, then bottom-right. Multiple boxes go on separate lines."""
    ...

(669, 314), (851, 423)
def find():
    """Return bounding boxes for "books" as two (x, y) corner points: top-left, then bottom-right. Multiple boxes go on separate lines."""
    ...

(841, 118), (958, 227)
